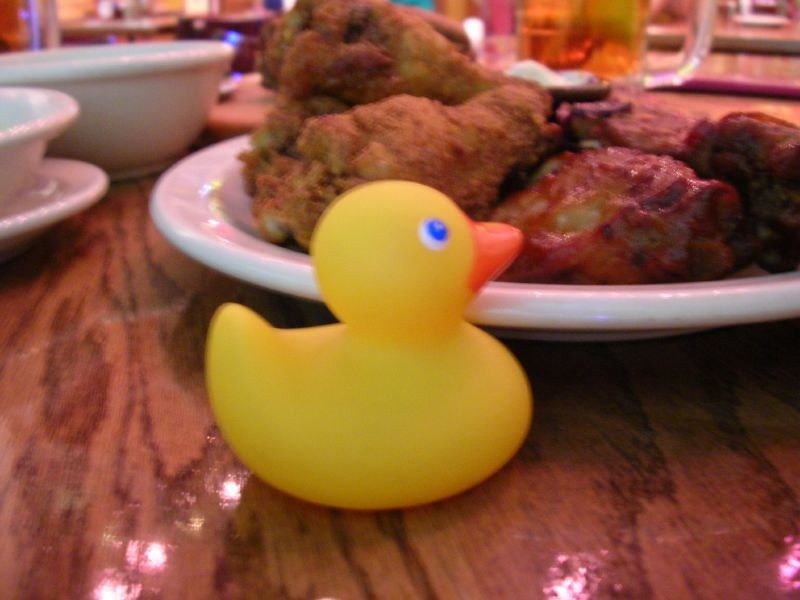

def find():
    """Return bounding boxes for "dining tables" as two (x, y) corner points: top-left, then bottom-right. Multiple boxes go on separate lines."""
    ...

(0, 55), (800, 598)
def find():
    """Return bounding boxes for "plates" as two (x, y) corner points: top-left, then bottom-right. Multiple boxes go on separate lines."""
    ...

(496, 70), (611, 99)
(147, 133), (800, 343)
(0, 157), (109, 263)
(204, 70), (281, 138)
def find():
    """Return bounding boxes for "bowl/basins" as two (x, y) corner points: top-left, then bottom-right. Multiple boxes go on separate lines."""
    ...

(0, 85), (79, 209)
(0, 37), (238, 181)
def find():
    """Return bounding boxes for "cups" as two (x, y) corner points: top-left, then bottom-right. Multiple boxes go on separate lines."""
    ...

(517, 0), (717, 99)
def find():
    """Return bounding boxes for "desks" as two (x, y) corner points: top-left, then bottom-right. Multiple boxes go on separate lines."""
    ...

(643, 17), (800, 54)
(42, 13), (178, 44)
